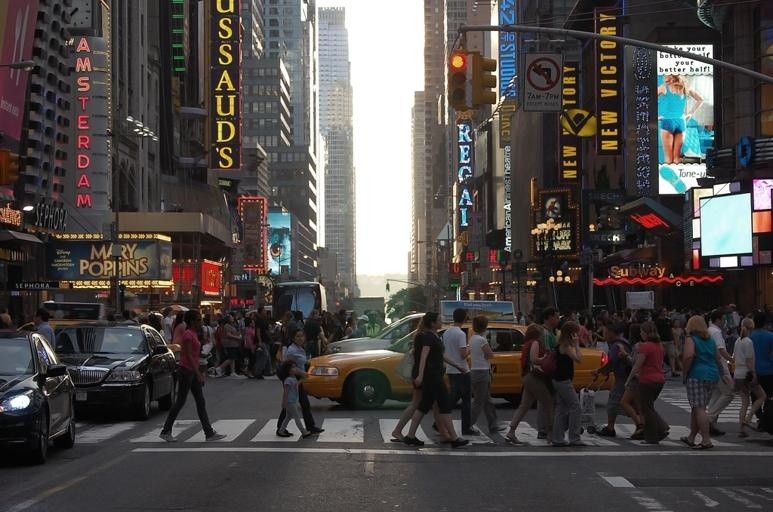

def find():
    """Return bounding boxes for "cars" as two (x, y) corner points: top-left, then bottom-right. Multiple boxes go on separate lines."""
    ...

(0, 327), (77, 464)
(301, 298), (612, 412)
(48, 323), (178, 417)
(321, 311), (427, 357)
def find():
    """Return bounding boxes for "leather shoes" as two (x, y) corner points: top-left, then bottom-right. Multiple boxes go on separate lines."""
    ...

(276, 429), (293, 438)
(432, 424), (440, 433)
(404, 435), (424, 446)
(302, 427), (325, 439)
(451, 438), (469, 448)
(462, 428), (480, 436)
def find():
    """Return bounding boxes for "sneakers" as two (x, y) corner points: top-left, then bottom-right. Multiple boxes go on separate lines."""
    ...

(631, 424), (670, 445)
(208, 367), (265, 380)
(672, 373), (681, 377)
(595, 426), (616, 437)
(537, 432), (547, 439)
(159, 430), (178, 442)
(495, 425), (507, 432)
(710, 427), (726, 436)
(205, 432), (228, 441)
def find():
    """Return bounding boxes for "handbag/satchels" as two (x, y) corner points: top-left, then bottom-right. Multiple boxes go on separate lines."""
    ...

(528, 360), (545, 377)
(540, 345), (574, 381)
(393, 352), (418, 384)
(578, 387), (596, 416)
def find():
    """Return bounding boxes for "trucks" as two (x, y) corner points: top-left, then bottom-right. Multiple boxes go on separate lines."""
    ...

(341, 295), (387, 329)
(37, 300), (107, 327)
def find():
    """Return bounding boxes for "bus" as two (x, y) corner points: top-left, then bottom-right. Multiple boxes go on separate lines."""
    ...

(270, 281), (331, 325)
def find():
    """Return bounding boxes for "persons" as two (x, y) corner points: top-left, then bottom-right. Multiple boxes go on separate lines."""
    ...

(391, 309), (507, 447)
(656, 75), (703, 163)
(276, 360), (312, 439)
(158, 309), (227, 443)
(0, 303), (77, 354)
(275, 327), (326, 436)
(506, 305), (772, 448)
(105, 304), (383, 382)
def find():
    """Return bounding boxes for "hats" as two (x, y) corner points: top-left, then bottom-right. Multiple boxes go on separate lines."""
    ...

(366, 310), (378, 318)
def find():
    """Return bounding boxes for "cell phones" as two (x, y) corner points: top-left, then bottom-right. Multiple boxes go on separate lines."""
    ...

(568, 330), (575, 339)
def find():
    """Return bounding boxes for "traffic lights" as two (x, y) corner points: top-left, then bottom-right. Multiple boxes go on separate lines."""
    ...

(446, 51), (470, 111)
(471, 50), (496, 107)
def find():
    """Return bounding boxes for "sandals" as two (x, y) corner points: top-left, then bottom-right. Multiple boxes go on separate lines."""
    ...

(680, 437), (696, 446)
(504, 433), (524, 444)
(741, 418), (758, 432)
(735, 431), (751, 438)
(693, 444), (714, 450)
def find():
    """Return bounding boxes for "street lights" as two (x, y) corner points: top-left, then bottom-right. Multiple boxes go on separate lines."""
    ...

(226, 223), (270, 303)
(409, 239), (452, 300)
(0, 60), (38, 74)
(276, 254), (308, 282)
(384, 279), (450, 303)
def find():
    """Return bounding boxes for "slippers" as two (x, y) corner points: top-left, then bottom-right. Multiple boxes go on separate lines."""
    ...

(390, 437), (405, 442)
(440, 440), (451, 444)
